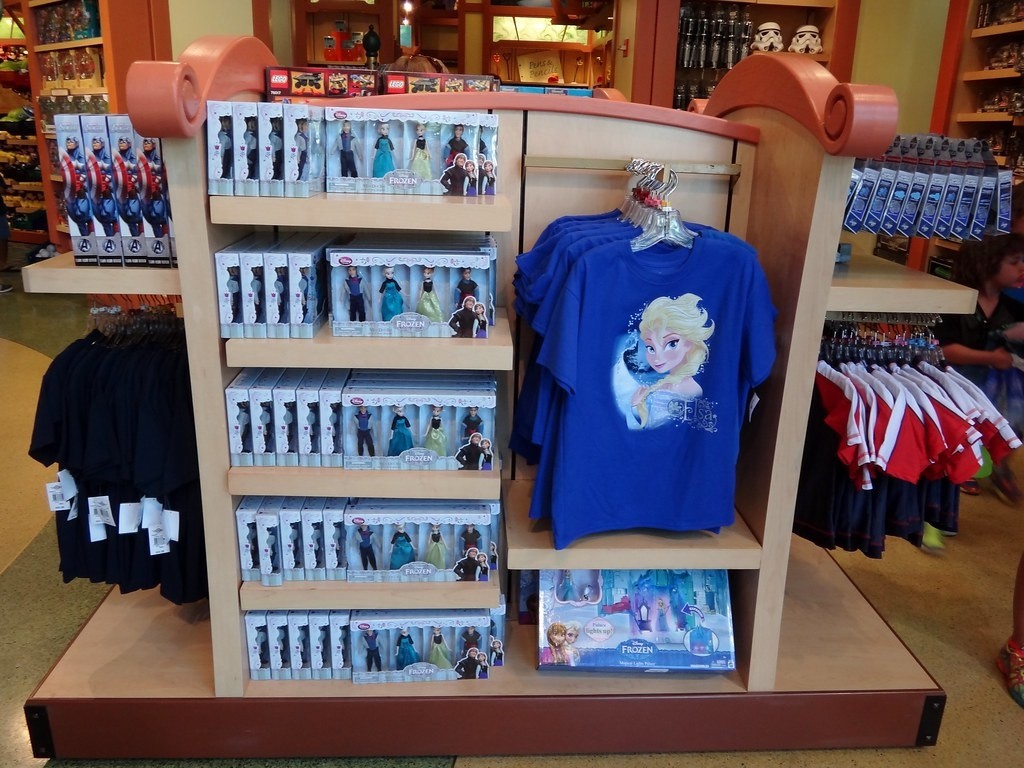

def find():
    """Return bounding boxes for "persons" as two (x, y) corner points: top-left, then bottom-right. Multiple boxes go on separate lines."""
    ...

(257, 615), (497, 676)
(556, 570), (581, 604)
(213, 113), (490, 186)
(218, 257), (480, 336)
(234, 396), (484, 457)
(930, 191), (1024, 707)
(241, 517), (481, 579)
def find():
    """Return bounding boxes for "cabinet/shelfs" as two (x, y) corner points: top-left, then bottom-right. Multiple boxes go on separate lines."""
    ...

(285, 3), (621, 105)
(672, 3), (833, 119)
(0, 1), (48, 245)
(21, 0), (122, 256)
(954, 4), (1023, 179)
(16, 42), (984, 695)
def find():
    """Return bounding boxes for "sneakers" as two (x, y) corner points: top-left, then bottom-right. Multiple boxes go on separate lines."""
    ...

(986, 469), (1019, 504)
(959, 477), (982, 494)
(996, 638), (1024, 709)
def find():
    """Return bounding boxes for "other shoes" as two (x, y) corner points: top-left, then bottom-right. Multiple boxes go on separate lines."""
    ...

(921, 522), (956, 552)
